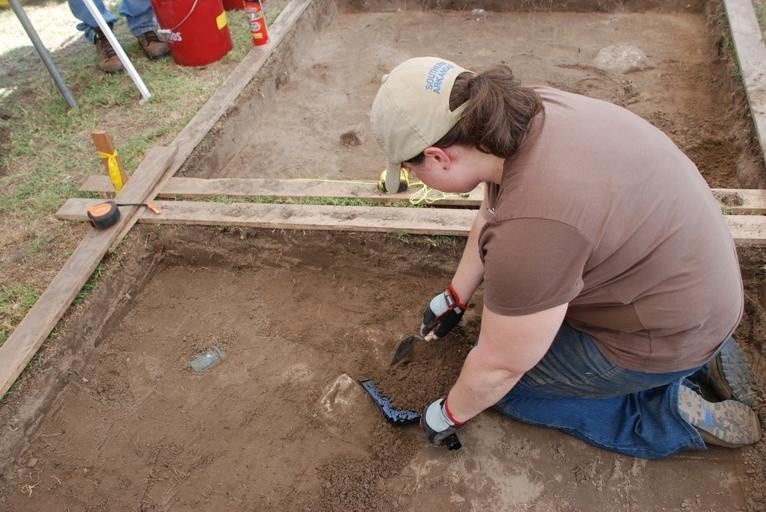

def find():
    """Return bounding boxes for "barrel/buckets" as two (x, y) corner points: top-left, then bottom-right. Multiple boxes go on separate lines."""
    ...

(149, 0), (233, 67)
(221, 0), (245, 11)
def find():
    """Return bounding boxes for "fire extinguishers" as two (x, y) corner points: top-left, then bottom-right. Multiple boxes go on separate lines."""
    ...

(246, 0), (269, 45)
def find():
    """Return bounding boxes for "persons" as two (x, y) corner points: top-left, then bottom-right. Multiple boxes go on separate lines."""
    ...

(66, 0), (170, 77)
(368, 53), (763, 465)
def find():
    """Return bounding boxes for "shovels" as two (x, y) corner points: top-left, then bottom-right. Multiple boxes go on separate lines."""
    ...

(391, 322), (441, 366)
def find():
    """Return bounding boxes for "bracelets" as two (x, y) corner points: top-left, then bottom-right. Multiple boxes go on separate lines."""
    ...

(442, 396), (465, 428)
(443, 286), (467, 319)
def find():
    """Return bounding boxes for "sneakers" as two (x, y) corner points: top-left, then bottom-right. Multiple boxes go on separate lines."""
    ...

(94, 22), (124, 73)
(137, 30), (170, 59)
(707, 336), (763, 422)
(677, 385), (762, 449)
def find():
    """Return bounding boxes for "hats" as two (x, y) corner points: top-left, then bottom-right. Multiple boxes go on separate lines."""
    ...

(369, 56), (480, 195)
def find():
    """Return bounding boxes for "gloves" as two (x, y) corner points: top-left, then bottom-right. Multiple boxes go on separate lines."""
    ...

(420, 285), (464, 342)
(419, 396), (462, 448)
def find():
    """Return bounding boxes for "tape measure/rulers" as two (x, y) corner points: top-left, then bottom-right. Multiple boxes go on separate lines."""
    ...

(87, 200), (160, 230)
